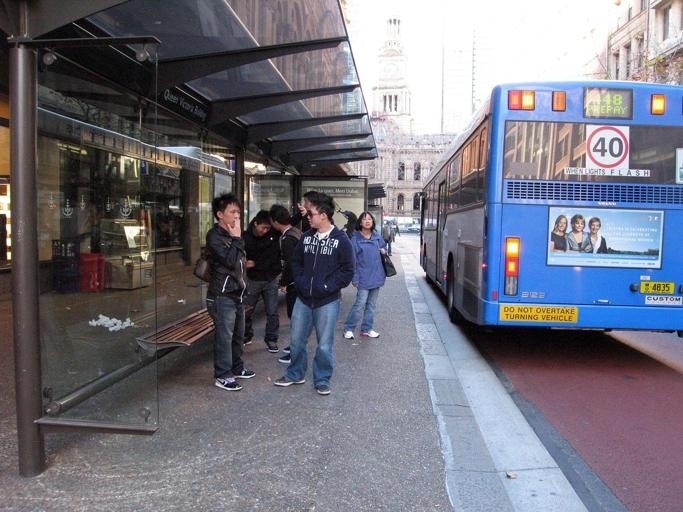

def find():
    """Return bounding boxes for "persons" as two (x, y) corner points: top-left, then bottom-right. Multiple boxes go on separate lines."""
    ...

(391, 220), (403, 243)
(344, 212), (388, 342)
(583, 217), (608, 252)
(240, 208), (283, 354)
(382, 219), (394, 256)
(567, 214), (590, 250)
(552, 214), (568, 252)
(296, 191), (319, 230)
(204, 189), (257, 392)
(270, 203), (306, 364)
(274, 191), (354, 396)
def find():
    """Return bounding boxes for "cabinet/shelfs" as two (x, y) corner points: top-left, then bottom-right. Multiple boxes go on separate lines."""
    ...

(101, 220), (155, 290)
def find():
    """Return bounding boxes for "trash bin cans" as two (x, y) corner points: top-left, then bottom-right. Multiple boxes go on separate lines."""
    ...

(81, 253), (105, 292)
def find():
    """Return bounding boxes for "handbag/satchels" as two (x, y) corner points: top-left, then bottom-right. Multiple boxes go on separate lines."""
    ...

(381, 254), (396, 277)
(193, 259), (208, 280)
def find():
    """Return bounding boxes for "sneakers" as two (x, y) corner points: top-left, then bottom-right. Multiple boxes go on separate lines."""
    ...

(360, 330), (379, 338)
(215, 377), (242, 390)
(244, 337), (252, 346)
(317, 385), (330, 394)
(267, 345), (305, 387)
(235, 368), (255, 378)
(343, 331), (354, 339)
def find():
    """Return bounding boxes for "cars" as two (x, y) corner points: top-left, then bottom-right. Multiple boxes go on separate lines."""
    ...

(396, 224), (420, 235)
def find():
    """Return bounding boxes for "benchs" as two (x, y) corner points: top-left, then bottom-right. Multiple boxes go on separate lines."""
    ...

(136, 302), (254, 353)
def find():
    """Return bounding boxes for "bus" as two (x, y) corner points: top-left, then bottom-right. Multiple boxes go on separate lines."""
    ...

(418, 80), (682, 342)
